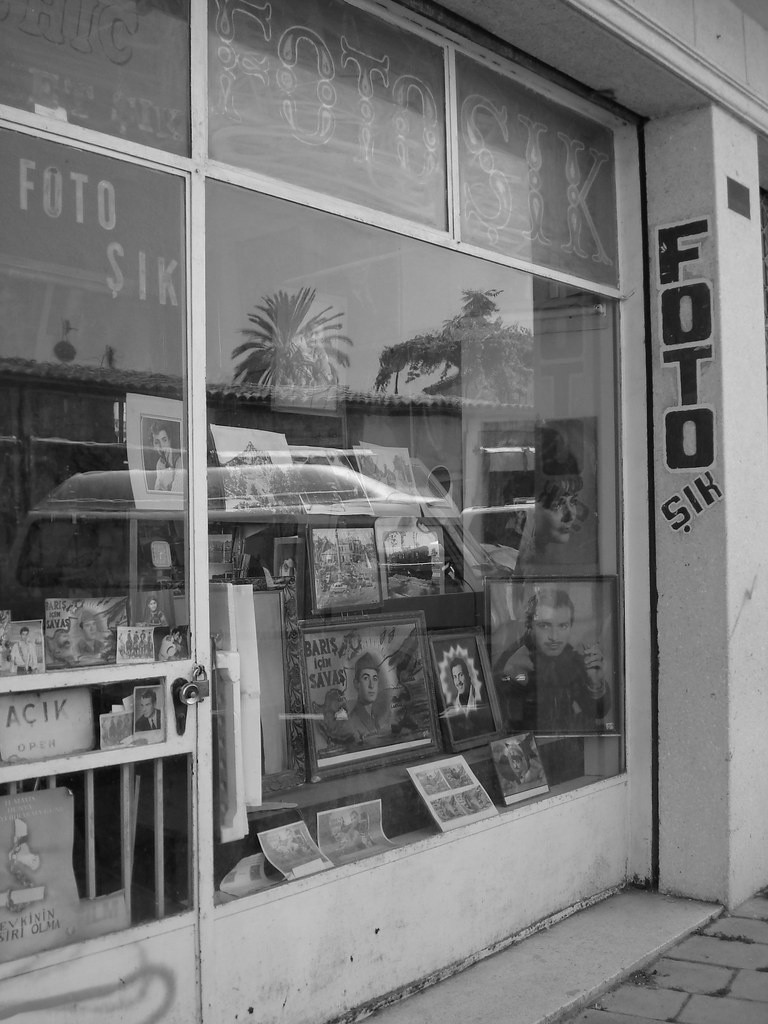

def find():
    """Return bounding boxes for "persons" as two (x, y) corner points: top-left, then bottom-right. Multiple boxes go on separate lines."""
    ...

(346, 652), (388, 737)
(135, 691), (161, 731)
(150, 422), (186, 494)
(501, 427), (582, 574)
(450, 658), (478, 712)
(508, 747), (524, 776)
(78, 611), (103, 655)
(10, 626), (37, 675)
(118, 598), (186, 658)
(495, 589), (611, 786)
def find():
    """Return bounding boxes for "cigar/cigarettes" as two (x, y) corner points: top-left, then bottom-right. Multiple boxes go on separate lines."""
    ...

(592, 641), (599, 649)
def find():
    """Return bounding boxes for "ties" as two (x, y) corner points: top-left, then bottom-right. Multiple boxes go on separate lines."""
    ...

(151, 719), (156, 730)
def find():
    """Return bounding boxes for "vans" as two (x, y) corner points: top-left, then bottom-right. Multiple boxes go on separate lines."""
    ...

(0, 468), (519, 628)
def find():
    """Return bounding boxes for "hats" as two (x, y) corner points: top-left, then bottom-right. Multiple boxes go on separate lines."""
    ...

(77, 611), (96, 629)
(355, 651), (379, 676)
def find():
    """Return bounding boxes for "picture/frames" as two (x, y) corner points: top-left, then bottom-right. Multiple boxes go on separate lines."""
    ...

(156, 524), (623, 799)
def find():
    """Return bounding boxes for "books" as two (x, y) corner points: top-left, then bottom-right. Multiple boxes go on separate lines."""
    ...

(405, 755), (499, 831)
